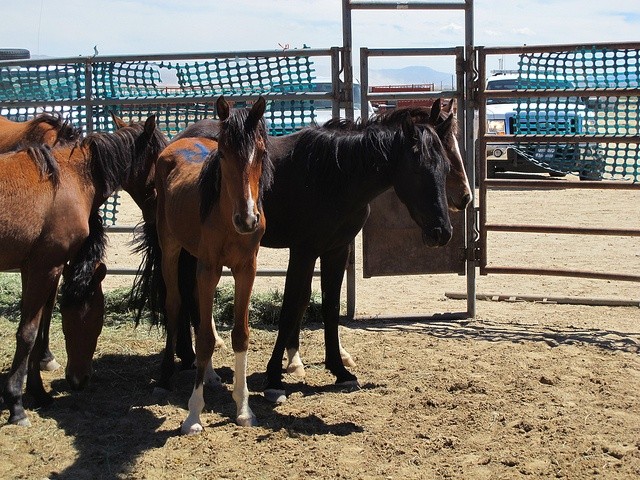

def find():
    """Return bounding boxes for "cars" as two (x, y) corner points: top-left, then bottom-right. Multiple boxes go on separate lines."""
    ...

(260, 79), (383, 134)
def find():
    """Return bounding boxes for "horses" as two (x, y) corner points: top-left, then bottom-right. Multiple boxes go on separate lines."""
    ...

(152, 96), (279, 436)
(191, 96), (473, 382)
(0, 109), (171, 426)
(0, 110), (110, 393)
(121, 109), (454, 405)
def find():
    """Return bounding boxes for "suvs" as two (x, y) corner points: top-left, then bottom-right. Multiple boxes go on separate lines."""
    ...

(0, 48), (120, 140)
(484, 67), (610, 183)
